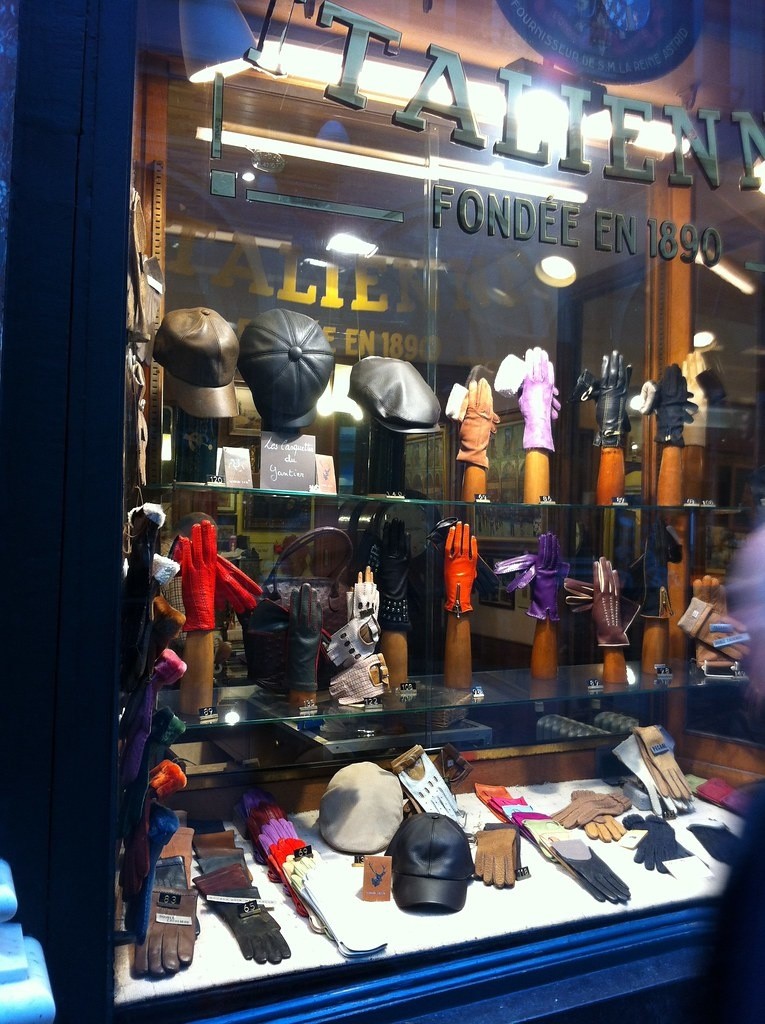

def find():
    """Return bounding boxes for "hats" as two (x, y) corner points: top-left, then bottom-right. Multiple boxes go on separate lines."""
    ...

(152, 308), (239, 417)
(384, 813), (474, 911)
(237, 309), (333, 427)
(319, 761), (403, 852)
(347, 356), (441, 433)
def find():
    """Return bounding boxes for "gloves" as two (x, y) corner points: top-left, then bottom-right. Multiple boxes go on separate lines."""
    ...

(370, 518), (752, 667)
(135, 786), (388, 982)
(174, 520), (324, 690)
(456, 347), (709, 469)
(390, 726), (765, 905)
(117, 186), (188, 947)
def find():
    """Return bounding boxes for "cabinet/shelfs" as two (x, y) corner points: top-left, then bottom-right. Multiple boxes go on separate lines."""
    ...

(131, 42), (765, 829)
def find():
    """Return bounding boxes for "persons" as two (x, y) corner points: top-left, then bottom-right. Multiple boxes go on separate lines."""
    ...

(690, 522), (765, 1024)
